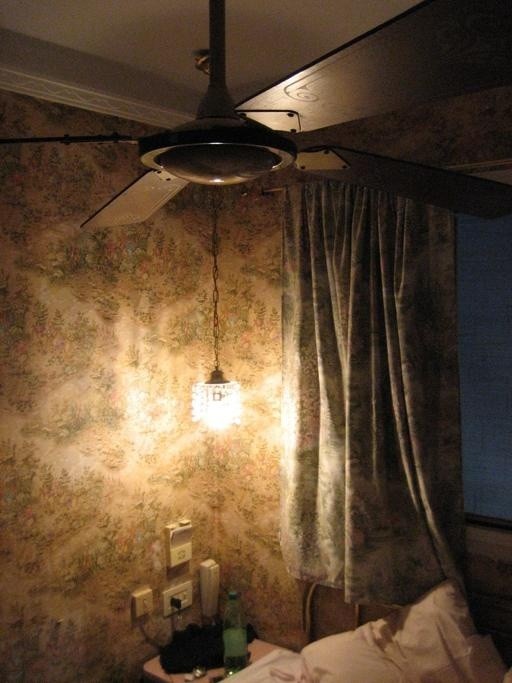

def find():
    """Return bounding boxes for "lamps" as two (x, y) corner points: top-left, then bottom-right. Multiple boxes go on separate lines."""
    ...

(189, 184), (246, 435)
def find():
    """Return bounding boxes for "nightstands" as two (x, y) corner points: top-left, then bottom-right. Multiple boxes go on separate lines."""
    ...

(142, 638), (285, 683)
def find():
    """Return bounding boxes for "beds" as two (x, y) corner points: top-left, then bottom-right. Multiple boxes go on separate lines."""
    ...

(218, 580), (512, 683)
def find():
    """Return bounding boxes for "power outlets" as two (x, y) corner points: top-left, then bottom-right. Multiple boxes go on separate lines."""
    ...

(160, 579), (193, 618)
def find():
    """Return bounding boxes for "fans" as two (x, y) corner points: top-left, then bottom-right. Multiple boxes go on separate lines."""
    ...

(0, 0), (512, 230)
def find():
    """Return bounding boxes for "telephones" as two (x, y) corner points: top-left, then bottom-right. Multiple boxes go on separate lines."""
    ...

(200, 559), (219, 618)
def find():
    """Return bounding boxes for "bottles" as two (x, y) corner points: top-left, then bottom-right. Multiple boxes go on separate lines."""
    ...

(222, 591), (250, 677)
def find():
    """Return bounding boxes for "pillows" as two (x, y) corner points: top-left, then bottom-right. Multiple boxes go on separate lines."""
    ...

(299, 577), (505, 683)
